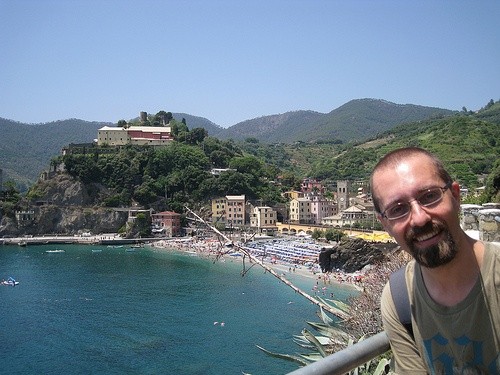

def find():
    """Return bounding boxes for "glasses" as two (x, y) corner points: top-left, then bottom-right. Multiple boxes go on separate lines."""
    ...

(381, 181), (452, 220)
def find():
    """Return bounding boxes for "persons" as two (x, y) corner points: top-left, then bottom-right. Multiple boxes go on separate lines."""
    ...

(370, 148), (500, 375)
(173, 236), (362, 298)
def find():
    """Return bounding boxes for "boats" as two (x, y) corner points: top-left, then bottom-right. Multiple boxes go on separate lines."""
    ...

(107, 245), (123, 249)
(130, 245), (140, 248)
(1, 277), (19, 285)
(127, 249), (135, 251)
(92, 249), (101, 252)
(46, 249), (65, 252)
(18, 241), (26, 246)
